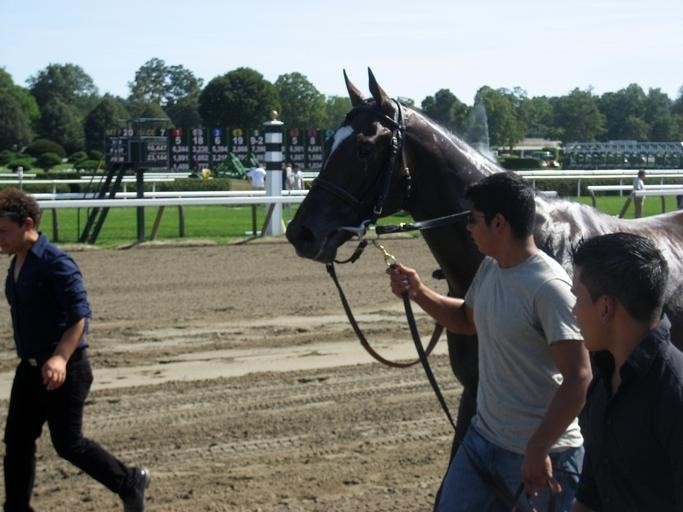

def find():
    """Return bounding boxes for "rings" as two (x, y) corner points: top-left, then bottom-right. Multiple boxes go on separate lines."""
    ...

(548, 476), (559, 487)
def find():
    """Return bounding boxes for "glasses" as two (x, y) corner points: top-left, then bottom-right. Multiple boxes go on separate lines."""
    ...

(467, 215), (486, 224)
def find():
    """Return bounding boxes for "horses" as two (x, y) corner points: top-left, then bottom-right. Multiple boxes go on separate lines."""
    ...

(284, 66), (683, 512)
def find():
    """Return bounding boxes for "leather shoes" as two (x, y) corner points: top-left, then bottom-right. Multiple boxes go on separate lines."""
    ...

(121, 466), (150, 512)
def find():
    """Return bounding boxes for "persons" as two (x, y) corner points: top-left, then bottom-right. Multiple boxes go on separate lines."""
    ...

(286, 162), (305, 225)
(384, 172), (591, 511)
(566, 226), (682, 512)
(633, 169), (646, 219)
(0, 184), (149, 512)
(246, 162), (266, 207)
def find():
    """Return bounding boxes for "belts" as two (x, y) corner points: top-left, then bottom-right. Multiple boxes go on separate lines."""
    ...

(24, 347), (87, 368)
